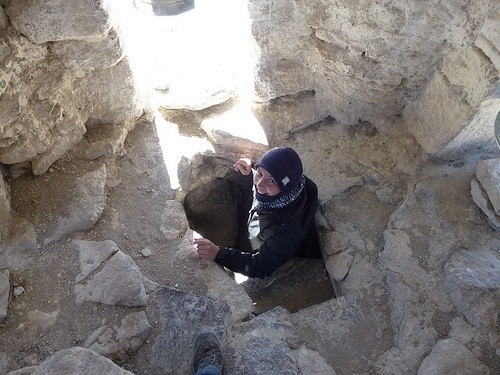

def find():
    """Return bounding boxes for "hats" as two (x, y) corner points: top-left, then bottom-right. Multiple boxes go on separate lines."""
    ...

(254, 145), (303, 196)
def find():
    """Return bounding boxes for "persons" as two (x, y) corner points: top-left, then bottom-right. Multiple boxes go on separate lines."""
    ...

(192, 330), (226, 375)
(193, 146), (320, 282)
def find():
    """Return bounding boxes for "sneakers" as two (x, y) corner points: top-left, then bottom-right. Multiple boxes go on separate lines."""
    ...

(189, 330), (225, 375)
